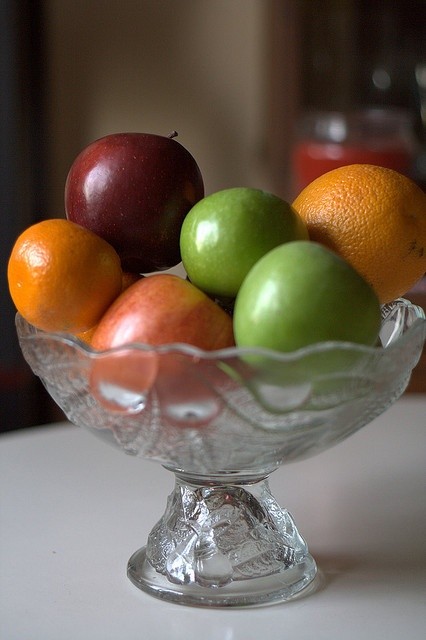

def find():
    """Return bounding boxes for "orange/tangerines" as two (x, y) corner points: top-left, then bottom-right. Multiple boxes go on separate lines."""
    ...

(8, 218), (122, 333)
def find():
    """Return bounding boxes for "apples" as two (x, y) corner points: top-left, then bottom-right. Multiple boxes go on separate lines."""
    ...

(180, 188), (311, 297)
(87, 275), (233, 429)
(64, 132), (204, 272)
(233, 239), (381, 371)
